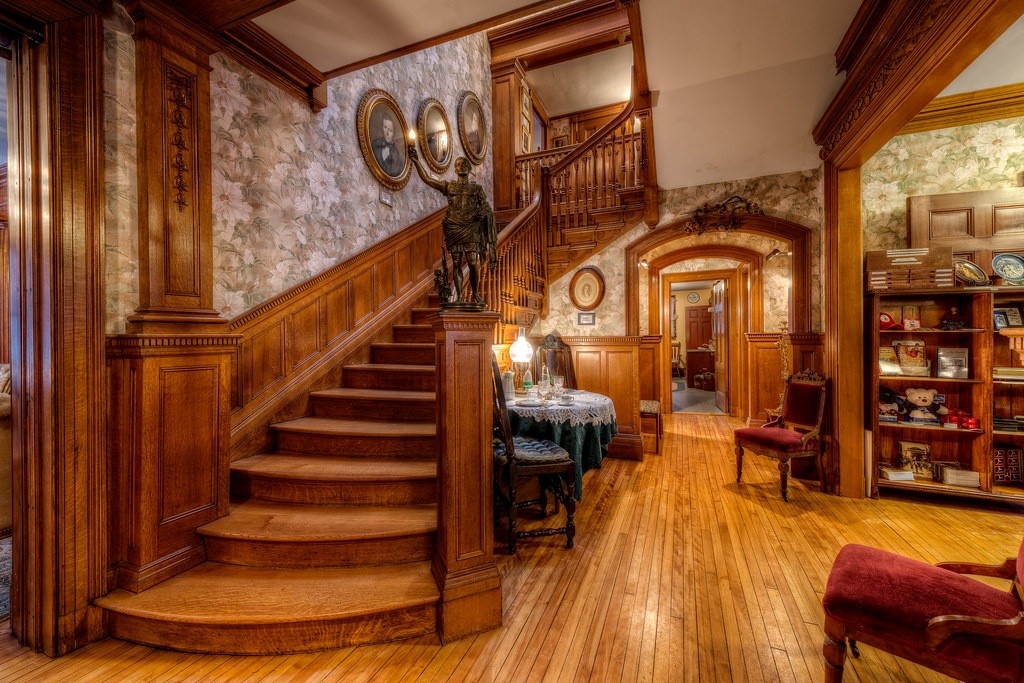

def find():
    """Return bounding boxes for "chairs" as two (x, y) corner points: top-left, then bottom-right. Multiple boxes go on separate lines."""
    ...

(821, 534), (1024, 683)
(491, 349), (577, 553)
(641, 399), (662, 454)
(734, 367), (829, 503)
(672, 342), (681, 377)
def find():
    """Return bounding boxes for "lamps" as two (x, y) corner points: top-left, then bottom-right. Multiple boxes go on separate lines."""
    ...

(508, 326), (533, 393)
(408, 125), (416, 147)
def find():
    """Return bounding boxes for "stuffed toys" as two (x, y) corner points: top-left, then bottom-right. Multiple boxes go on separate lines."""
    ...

(878, 386), (948, 424)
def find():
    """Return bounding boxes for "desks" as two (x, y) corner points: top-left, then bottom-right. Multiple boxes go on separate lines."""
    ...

(493, 389), (619, 503)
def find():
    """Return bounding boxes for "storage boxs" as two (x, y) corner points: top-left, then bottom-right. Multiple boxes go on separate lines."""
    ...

(867, 269), (957, 290)
(865, 245), (954, 270)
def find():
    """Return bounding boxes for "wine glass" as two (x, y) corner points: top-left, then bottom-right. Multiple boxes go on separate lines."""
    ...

(523, 375), (532, 396)
(538, 381), (550, 400)
(554, 375), (564, 395)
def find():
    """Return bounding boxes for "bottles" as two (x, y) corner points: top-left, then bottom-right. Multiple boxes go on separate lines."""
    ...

(501, 370), (516, 401)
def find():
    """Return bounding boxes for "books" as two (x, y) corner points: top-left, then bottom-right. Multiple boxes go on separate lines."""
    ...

(878, 440), (981, 487)
(992, 415), (1024, 432)
(878, 340), (970, 380)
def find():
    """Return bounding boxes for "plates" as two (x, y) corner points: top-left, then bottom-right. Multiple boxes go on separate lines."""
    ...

(515, 399), (544, 406)
(992, 253), (1024, 281)
(952, 259), (989, 286)
(557, 402), (574, 405)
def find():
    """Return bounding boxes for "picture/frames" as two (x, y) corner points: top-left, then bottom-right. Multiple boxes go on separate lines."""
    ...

(523, 87), (531, 122)
(567, 266), (605, 310)
(355, 87), (414, 189)
(418, 96), (453, 173)
(456, 90), (487, 165)
(522, 124), (531, 155)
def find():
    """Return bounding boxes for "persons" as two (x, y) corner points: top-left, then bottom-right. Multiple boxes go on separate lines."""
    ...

(433, 260), (451, 304)
(407, 144), (499, 305)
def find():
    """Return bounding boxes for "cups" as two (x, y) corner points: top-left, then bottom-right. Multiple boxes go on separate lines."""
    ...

(561, 395), (575, 403)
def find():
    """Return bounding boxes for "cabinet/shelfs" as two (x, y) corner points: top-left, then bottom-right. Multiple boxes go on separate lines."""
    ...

(872, 289), (1024, 506)
